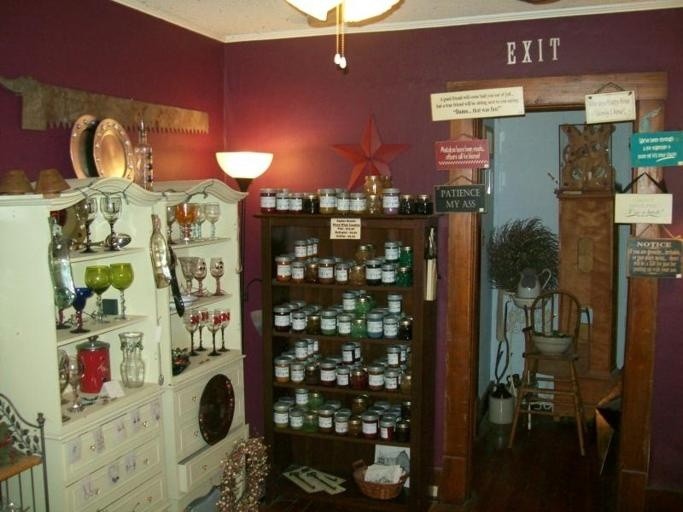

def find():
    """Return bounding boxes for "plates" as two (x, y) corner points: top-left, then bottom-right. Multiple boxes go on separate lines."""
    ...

(68, 112), (135, 185)
(198, 373), (237, 446)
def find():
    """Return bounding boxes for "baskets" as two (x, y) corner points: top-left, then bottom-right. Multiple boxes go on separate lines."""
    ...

(351, 460), (411, 499)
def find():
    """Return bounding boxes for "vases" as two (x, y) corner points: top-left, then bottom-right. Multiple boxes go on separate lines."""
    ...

(118, 332), (146, 388)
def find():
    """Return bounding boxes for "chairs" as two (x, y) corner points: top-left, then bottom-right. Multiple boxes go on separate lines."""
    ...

(507, 290), (587, 457)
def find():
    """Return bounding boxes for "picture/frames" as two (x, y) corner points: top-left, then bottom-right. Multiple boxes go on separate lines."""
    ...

(483, 125), (494, 159)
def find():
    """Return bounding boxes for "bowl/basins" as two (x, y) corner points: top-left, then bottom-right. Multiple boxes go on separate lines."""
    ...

(0, 168), (35, 195)
(530, 335), (572, 357)
(34, 167), (70, 195)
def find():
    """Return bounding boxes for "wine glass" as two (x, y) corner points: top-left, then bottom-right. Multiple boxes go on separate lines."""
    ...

(178, 255), (225, 297)
(98, 198), (124, 252)
(58, 366), (72, 422)
(184, 306), (232, 357)
(167, 201), (220, 243)
(72, 198), (97, 255)
(62, 357), (87, 412)
(55, 262), (133, 334)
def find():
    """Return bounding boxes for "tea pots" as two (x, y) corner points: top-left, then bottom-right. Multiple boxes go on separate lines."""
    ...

(515, 267), (551, 300)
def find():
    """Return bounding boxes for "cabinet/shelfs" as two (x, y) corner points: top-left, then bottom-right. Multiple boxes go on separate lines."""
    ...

(0, 394), (49, 512)
(253, 210), (445, 511)
(0, 174), (171, 512)
(152, 177), (250, 512)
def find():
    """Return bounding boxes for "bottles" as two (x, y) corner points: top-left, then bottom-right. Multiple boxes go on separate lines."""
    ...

(134, 121), (154, 190)
(76, 335), (111, 393)
(148, 214), (173, 290)
(47, 216), (78, 309)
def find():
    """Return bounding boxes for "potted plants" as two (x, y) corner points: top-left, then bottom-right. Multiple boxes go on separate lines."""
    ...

(530, 329), (574, 356)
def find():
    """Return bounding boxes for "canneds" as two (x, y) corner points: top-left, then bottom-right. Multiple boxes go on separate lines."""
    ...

(260, 174), (433, 441)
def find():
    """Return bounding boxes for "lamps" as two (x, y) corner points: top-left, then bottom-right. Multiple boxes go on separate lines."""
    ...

(214, 150), (274, 354)
(285, 0), (401, 69)
